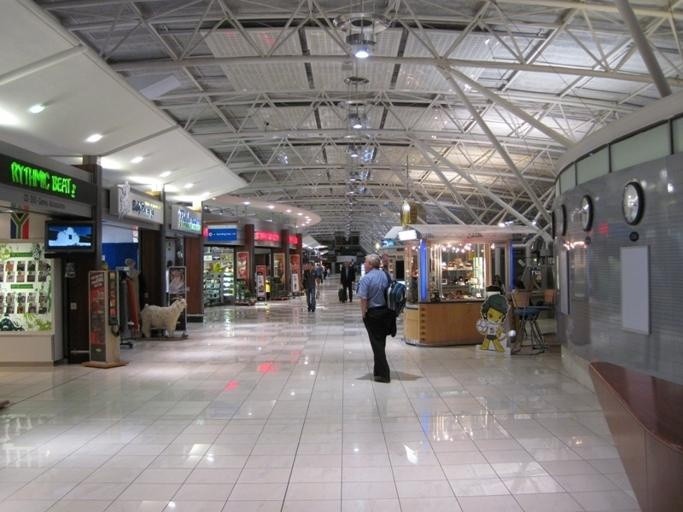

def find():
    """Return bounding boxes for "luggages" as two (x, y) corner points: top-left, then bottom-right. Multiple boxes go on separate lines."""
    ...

(338, 288), (347, 301)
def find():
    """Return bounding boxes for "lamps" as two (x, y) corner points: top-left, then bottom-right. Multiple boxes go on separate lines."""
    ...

(351, 175), (356, 181)
(352, 43), (369, 59)
(350, 148), (357, 157)
(349, 112), (364, 129)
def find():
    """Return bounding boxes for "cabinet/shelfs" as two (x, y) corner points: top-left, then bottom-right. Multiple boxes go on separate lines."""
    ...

(203, 246), (234, 307)
(428, 240), (487, 302)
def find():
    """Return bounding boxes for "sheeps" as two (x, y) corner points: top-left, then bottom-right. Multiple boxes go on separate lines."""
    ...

(140, 296), (188, 338)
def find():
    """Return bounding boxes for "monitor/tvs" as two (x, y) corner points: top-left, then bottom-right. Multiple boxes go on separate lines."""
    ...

(45, 221), (95, 252)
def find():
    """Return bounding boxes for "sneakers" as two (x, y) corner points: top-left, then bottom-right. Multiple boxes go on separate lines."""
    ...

(373, 370), (390, 383)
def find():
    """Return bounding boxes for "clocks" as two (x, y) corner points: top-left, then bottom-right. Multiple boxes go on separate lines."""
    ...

(579, 195), (593, 231)
(622, 183), (643, 226)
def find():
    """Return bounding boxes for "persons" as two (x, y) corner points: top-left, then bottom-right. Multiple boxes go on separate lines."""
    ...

(476, 294), (509, 352)
(303, 261), (355, 312)
(356, 255), (396, 382)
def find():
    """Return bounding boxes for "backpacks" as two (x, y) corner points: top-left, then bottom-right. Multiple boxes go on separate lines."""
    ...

(386, 281), (407, 312)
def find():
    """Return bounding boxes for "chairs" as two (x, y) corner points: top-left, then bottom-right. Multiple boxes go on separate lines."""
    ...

(511, 288), (550, 354)
